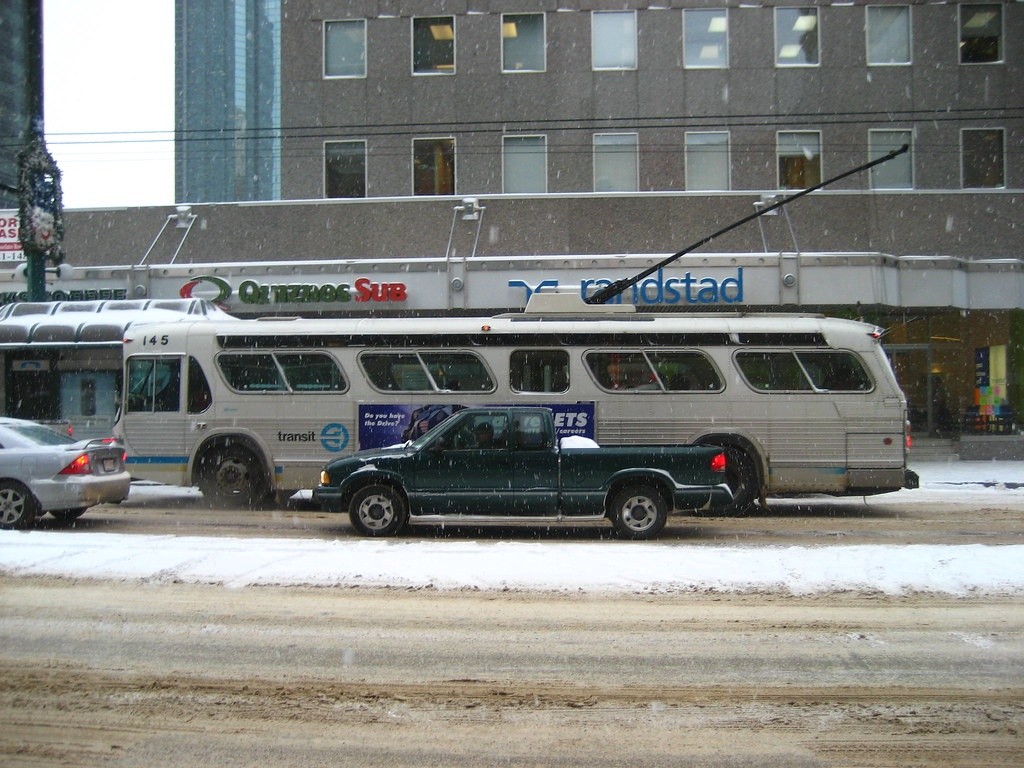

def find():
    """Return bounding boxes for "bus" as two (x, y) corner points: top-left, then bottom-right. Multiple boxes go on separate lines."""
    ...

(0, 292), (920, 512)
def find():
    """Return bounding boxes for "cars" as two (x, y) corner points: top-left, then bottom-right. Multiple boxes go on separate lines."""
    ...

(0, 416), (132, 531)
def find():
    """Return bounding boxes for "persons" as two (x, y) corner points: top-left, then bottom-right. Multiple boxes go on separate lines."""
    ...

(146, 364), (661, 412)
(476, 422), (494, 448)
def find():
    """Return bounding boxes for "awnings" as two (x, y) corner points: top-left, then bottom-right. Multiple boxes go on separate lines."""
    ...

(0, 299), (217, 349)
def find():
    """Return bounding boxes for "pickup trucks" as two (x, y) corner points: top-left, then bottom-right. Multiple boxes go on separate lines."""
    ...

(312, 406), (735, 541)
